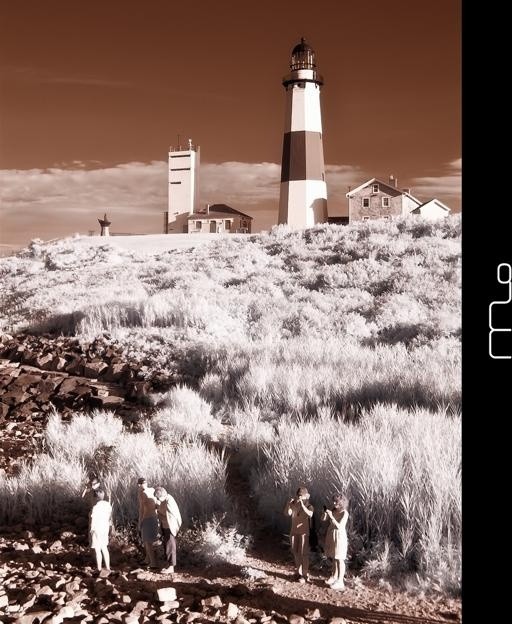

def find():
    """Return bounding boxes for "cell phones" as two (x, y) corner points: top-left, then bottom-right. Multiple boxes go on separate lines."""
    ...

(323, 506), (327, 512)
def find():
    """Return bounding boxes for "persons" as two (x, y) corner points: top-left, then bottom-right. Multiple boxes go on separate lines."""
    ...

(317, 492), (350, 592)
(86, 487), (116, 575)
(283, 484), (314, 585)
(150, 487), (183, 574)
(81, 477), (101, 565)
(134, 475), (159, 568)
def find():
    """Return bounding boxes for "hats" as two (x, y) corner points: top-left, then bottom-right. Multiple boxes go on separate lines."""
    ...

(138, 478), (145, 484)
(297, 488), (307, 496)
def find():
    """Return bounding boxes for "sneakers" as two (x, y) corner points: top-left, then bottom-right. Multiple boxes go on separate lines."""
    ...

(325, 577), (344, 588)
